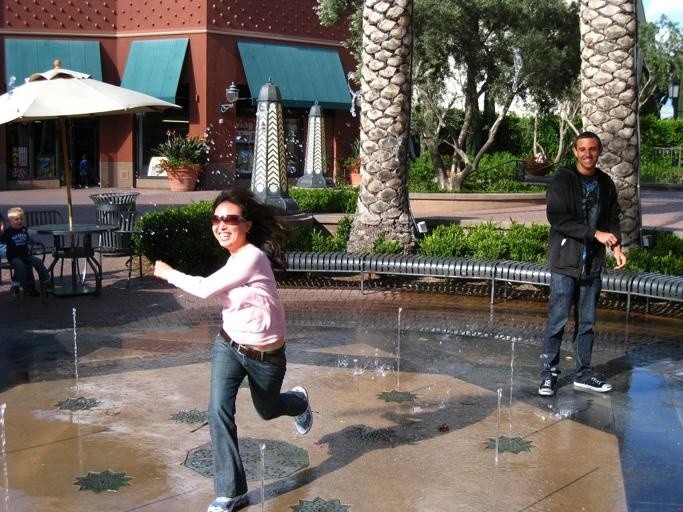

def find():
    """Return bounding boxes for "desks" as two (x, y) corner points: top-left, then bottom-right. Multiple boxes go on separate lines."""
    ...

(24, 224), (119, 294)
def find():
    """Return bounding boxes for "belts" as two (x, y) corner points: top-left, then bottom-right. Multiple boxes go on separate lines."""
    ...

(219, 327), (286, 366)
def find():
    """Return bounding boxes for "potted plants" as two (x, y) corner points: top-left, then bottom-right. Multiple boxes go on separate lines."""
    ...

(344, 158), (363, 190)
(149, 126), (213, 192)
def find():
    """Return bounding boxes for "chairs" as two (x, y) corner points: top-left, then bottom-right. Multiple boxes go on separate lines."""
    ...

(23, 210), (65, 279)
(98, 209), (143, 288)
(0, 214), (46, 282)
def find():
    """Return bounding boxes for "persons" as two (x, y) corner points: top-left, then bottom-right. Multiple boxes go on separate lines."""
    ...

(78, 154), (89, 189)
(0, 206), (52, 297)
(538, 131), (627, 396)
(155, 187), (313, 512)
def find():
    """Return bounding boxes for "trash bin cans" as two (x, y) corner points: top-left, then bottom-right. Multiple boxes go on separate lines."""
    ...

(88, 190), (141, 259)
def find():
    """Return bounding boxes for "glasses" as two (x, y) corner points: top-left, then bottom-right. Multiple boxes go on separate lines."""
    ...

(207, 213), (247, 226)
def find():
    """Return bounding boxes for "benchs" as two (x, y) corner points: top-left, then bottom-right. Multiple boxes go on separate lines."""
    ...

(362, 252), (508, 305)
(491, 258), (650, 317)
(271, 251), (369, 294)
(627, 272), (683, 318)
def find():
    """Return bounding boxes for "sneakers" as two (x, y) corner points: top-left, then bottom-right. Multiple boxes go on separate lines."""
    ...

(292, 386), (314, 435)
(41, 282), (55, 290)
(538, 380), (555, 396)
(573, 376), (612, 393)
(8, 285), (20, 300)
(207, 491), (251, 512)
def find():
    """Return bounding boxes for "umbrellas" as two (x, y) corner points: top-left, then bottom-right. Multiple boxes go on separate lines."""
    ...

(0, 58), (183, 285)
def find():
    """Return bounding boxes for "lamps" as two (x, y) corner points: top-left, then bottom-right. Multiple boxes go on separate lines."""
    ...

(219, 81), (240, 112)
